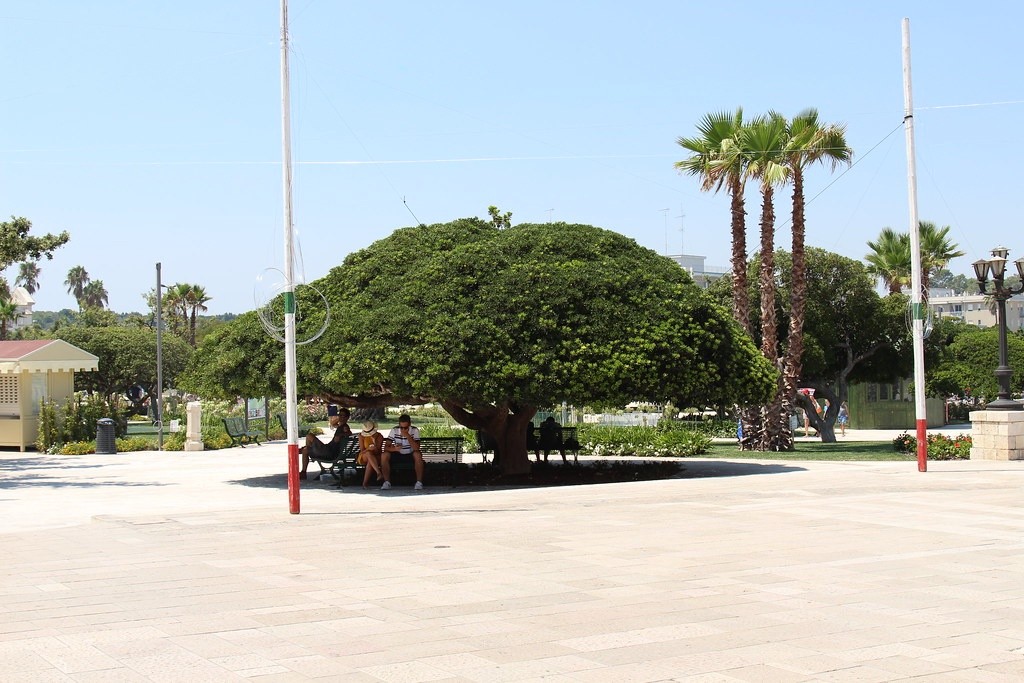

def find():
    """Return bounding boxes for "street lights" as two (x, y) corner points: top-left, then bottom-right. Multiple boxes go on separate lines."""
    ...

(970, 244), (1024, 411)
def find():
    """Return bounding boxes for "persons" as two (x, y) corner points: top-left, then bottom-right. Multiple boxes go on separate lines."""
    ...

(358, 420), (384, 489)
(538, 417), (571, 467)
(381, 414), (424, 490)
(527, 421), (542, 464)
(326, 403), (339, 430)
(299, 408), (352, 479)
(801, 389), (849, 436)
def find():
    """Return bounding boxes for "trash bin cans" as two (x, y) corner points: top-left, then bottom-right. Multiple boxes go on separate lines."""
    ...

(328, 405), (337, 416)
(95, 418), (117, 454)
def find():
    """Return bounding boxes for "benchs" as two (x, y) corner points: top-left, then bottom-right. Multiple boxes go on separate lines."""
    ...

(274, 414), (314, 438)
(221, 417), (264, 448)
(311, 433), (464, 489)
(475, 427), (583, 463)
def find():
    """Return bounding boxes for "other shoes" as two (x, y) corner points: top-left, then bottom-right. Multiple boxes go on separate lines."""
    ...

(362, 484), (371, 490)
(376, 473), (384, 481)
(299, 473), (307, 480)
(298, 445), (306, 454)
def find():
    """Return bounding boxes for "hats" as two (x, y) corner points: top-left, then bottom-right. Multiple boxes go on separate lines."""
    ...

(360, 421), (377, 437)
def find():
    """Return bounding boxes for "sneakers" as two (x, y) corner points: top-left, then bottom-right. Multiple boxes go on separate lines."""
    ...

(414, 481), (423, 490)
(381, 481), (392, 490)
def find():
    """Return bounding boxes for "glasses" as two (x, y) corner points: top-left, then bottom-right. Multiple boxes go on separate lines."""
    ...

(399, 426), (408, 430)
